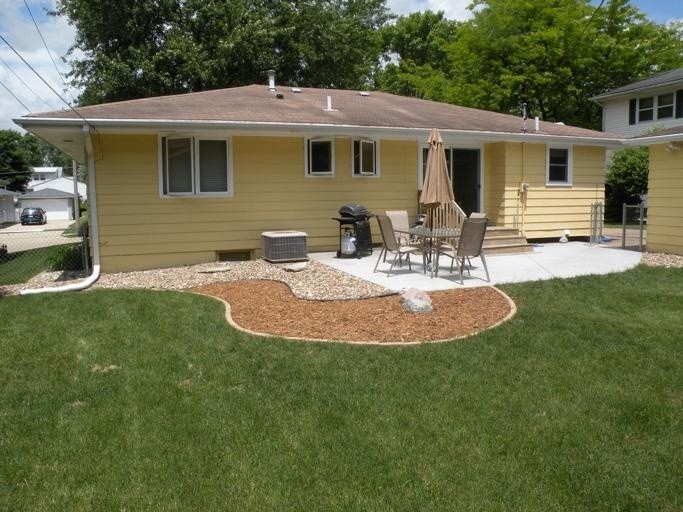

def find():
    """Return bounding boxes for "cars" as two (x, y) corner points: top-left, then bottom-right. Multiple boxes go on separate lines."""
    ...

(19, 207), (47, 225)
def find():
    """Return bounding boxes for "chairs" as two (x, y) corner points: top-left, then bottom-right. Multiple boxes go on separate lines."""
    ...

(436, 210), (489, 274)
(436, 216), (491, 286)
(383, 210), (428, 267)
(373, 213), (420, 279)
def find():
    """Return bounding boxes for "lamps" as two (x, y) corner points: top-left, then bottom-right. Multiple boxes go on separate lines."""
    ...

(662, 141), (680, 153)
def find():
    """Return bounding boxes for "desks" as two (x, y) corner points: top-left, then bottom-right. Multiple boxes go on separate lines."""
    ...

(392, 226), (462, 279)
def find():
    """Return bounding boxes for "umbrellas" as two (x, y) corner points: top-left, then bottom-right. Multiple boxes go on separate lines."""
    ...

(419, 128), (455, 264)
